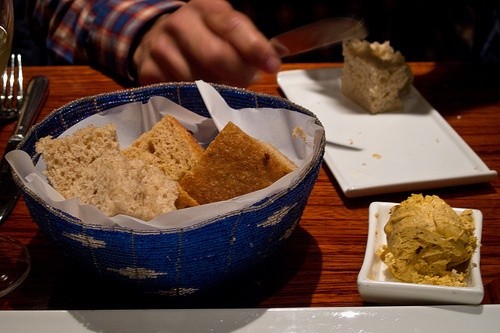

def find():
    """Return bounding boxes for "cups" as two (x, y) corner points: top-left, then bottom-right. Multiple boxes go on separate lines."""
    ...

(0, 0), (15, 75)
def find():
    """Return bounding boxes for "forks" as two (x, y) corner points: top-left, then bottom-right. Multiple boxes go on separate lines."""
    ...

(2, 53), (23, 119)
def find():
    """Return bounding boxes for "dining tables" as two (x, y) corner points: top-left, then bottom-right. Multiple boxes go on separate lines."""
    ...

(0, 61), (500, 311)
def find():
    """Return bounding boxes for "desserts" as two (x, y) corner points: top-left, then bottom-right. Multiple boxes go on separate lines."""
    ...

(376, 194), (478, 287)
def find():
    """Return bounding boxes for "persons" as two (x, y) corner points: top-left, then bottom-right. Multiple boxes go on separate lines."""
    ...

(33, 0), (396, 89)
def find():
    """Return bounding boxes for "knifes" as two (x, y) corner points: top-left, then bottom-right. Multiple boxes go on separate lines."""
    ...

(0, 73), (60, 223)
(267, 17), (368, 58)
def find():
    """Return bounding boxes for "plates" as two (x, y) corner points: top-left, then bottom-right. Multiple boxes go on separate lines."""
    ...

(275, 67), (496, 200)
(358, 201), (485, 306)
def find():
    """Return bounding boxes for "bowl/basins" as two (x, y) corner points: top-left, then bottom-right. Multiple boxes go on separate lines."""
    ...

(12, 81), (328, 291)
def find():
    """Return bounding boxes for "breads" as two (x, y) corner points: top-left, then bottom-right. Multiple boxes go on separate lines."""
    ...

(340, 39), (413, 115)
(64, 149), (179, 220)
(121, 114), (204, 182)
(174, 122), (300, 212)
(40, 123), (122, 191)
